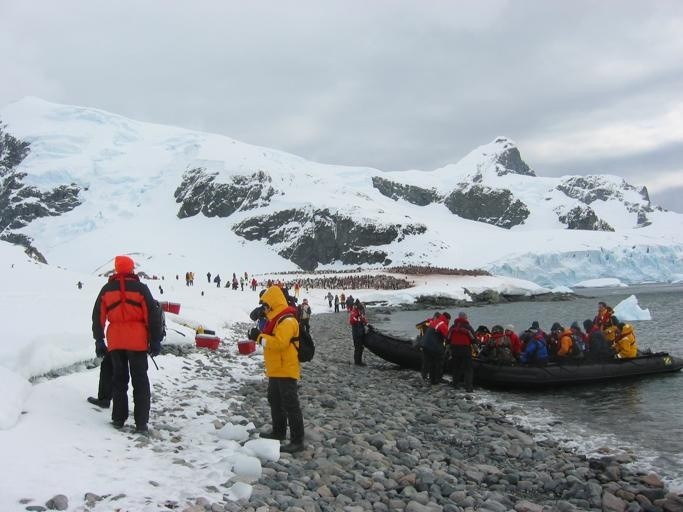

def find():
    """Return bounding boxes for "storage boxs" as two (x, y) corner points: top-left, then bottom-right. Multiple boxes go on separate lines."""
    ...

(194, 333), (220, 350)
(160, 300), (168, 314)
(170, 301), (181, 314)
(237, 339), (257, 353)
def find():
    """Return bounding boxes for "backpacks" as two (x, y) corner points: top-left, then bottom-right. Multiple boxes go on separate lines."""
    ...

(277, 315), (315, 363)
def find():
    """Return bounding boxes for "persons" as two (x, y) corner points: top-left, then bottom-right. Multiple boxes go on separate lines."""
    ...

(593, 302), (620, 330)
(531, 320), (548, 344)
(87, 351), (113, 409)
(415, 312), (441, 350)
(519, 326), (548, 367)
(91, 255), (160, 431)
(294, 282), (299, 297)
(301, 298), (311, 334)
(350, 304), (369, 366)
(504, 324), (522, 353)
(549, 322), (561, 347)
(569, 321), (586, 353)
(447, 311), (477, 393)
(556, 327), (572, 356)
(325, 292), (365, 315)
(422, 312), (451, 383)
(611, 323), (637, 358)
(583, 319), (608, 362)
(185, 271), (257, 291)
(247, 285), (304, 453)
(478, 325), (517, 367)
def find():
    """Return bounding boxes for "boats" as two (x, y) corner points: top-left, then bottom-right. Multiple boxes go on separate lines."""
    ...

(364, 328), (683, 383)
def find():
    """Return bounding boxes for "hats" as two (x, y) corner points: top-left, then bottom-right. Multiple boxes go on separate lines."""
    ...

(505, 324), (514, 331)
(525, 326), (537, 333)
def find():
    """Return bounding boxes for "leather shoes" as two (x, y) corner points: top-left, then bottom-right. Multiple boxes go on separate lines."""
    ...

(136, 424), (148, 432)
(111, 421), (124, 428)
(280, 441), (304, 453)
(87, 396), (110, 408)
(259, 432), (286, 440)
